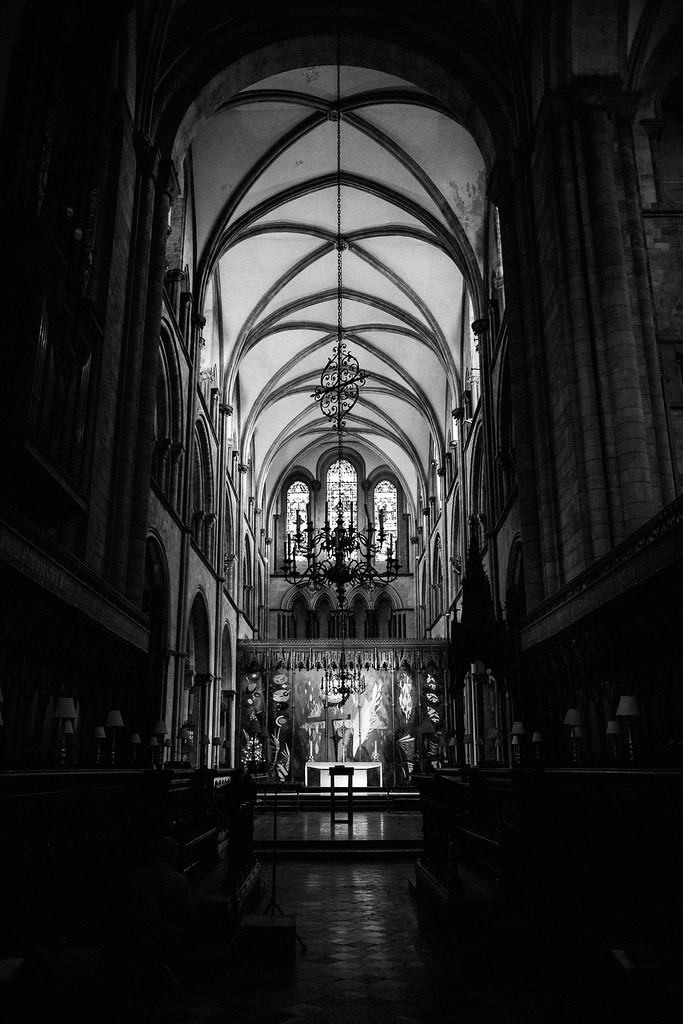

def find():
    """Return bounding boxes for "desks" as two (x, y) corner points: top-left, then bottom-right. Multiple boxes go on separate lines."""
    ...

(328, 767), (355, 788)
(256, 783), (305, 805)
(304, 762), (383, 787)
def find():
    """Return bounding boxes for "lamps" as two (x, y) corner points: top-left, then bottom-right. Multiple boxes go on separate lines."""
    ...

(617, 695), (641, 759)
(128, 733), (141, 760)
(448, 736), (458, 745)
(222, 740), (230, 767)
(267, 70), (414, 608)
(149, 737), (159, 765)
(54, 698), (78, 767)
(152, 720), (168, 741)
(177, 728), (189, 764)
(511, 735), (519, 762)
(463, 735), (474, 764)
(561, 708), (584, 762)
(163, 739), (172, 760)
(531, 732), (543, 759)
(511, 721), (525, 734)
(213, 738), (221, 763)
(94, 727), (105, 765)
(319, 629), (367, 697)
(475, 738), (484, 746)
(604, 722), (621, 758)
(200, 735), (210, 745)
(104, 710), (124, 763)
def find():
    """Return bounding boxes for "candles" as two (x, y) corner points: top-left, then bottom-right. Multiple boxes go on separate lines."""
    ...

(310, 741), (313, 756)
(375, 740), (377, 756)
